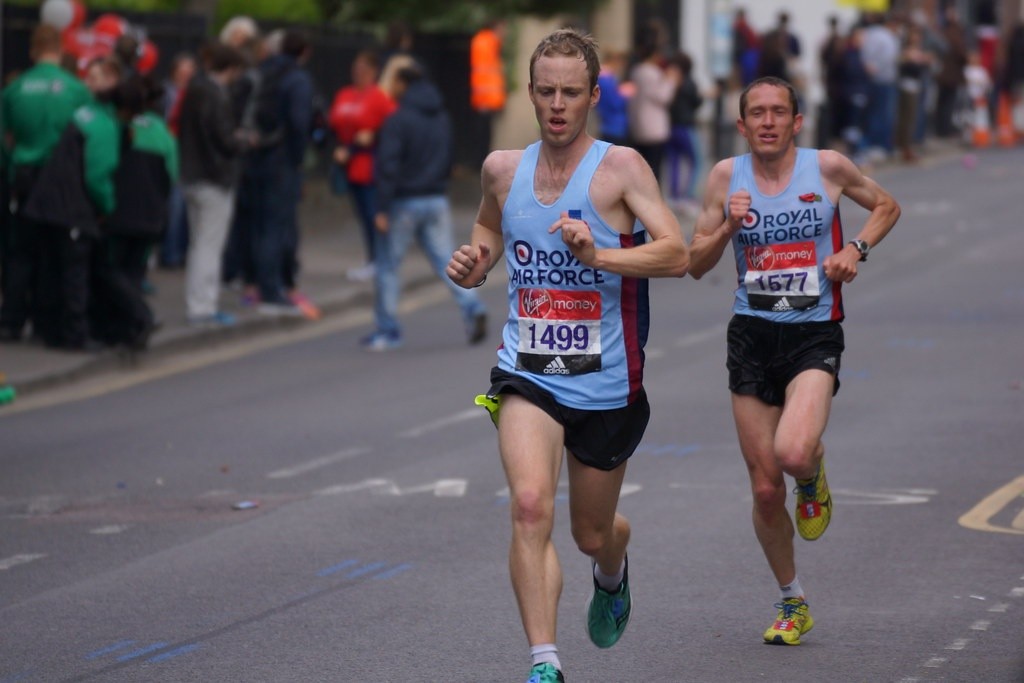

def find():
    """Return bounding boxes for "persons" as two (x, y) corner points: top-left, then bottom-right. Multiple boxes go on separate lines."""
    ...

(689, 78), (902, 646)
(592, 45), (705, 212)
(732, 0), (1024, 163)
(447, 29), (689, 683)
(0, 0), (510, 347)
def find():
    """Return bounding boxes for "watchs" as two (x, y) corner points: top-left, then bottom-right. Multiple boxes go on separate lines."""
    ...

(849, 240), (869, 261)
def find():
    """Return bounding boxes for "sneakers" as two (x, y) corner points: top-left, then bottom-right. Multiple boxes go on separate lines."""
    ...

(792, 459), (832, 541)
(525, 662), (564, 683)
(762, 596), (814, 646)
(584, 549), (632, 649)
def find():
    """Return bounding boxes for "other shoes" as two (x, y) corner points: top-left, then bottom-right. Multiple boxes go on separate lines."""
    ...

(462, 302), (488, 343)
(359, 328), (401, 350)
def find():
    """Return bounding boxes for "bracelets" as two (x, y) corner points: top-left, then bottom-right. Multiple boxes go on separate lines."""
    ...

(473, 274), (487, 287)
